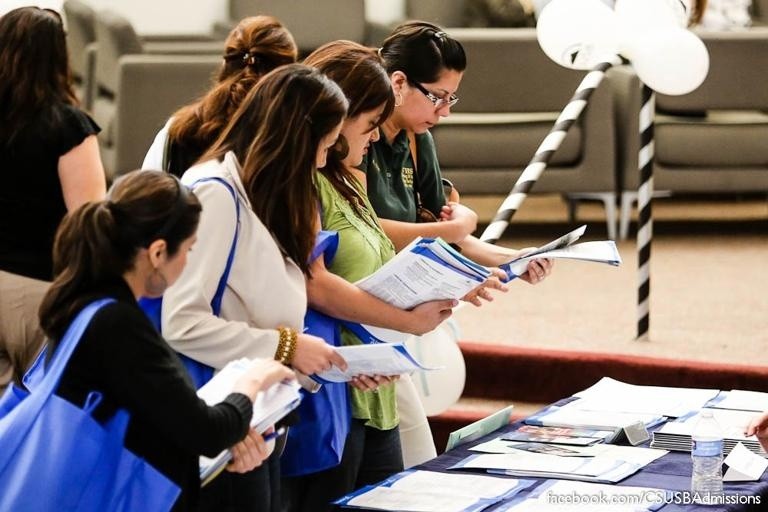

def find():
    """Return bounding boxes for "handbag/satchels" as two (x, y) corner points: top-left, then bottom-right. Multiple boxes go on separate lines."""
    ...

(138, 177), (240, 390)
(280, 230), (350, 479)
(1, 297), (182, 511)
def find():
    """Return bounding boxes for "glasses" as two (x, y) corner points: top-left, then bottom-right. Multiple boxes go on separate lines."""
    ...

(412, 81), (459, 108)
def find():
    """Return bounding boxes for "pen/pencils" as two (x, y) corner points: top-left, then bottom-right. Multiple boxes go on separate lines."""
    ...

(228, 427), (286, 465)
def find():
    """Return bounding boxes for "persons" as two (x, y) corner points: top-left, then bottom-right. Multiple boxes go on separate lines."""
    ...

(142, 13), (301, 181)
(35, 167), (300, 511)
(345, 20), (556, 470)
(0, 4), (108, 401)
(130, 61), (401, 510)
(276, 37), (511, 511)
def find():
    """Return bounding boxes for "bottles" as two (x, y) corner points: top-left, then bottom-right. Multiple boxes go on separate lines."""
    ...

(690, 406), (727, 507)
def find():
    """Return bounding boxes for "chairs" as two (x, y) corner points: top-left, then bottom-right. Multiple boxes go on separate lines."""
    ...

(62, 0), (768, 239)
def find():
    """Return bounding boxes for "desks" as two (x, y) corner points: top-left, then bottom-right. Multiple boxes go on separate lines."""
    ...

(328, 377), (768, 512)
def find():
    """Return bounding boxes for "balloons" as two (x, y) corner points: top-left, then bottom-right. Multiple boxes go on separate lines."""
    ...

(629, 26), (712, 98)
(612, 0), (689, 61)
(534, 0), (622, 73)
